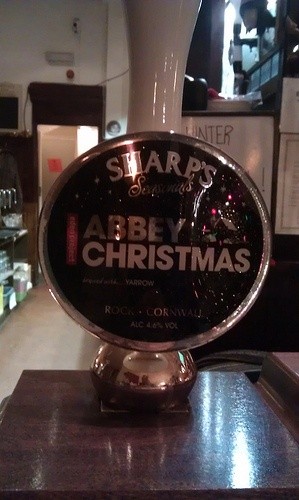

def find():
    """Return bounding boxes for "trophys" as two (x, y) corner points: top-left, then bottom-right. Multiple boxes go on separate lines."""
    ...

(34, 130), (273, 415)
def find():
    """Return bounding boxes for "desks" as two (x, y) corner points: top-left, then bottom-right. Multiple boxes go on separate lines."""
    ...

(0, 368), (298, 499)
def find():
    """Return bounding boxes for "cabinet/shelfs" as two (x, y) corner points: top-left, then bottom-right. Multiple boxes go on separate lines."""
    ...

(0, 227), (32, 327)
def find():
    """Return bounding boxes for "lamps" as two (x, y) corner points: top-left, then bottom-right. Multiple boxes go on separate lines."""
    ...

(239, 0), (275, 36)
